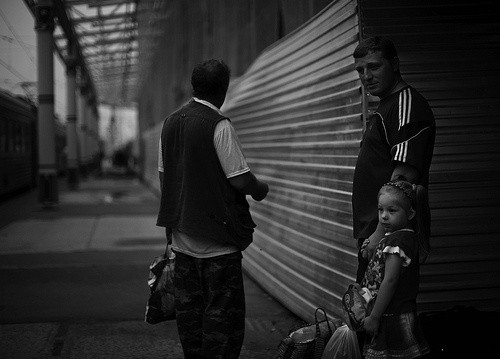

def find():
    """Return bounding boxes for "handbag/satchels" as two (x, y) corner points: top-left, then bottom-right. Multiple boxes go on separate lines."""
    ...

(144, 241), (178, 326)
(275, 308), (343, 359)
(342, 282), (378, 331)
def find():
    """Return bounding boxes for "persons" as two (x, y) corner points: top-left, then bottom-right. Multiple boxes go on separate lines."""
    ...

(352, 35), (436, 358)
(360, 181), (431, 358)
(156, 59), (269, 359)
